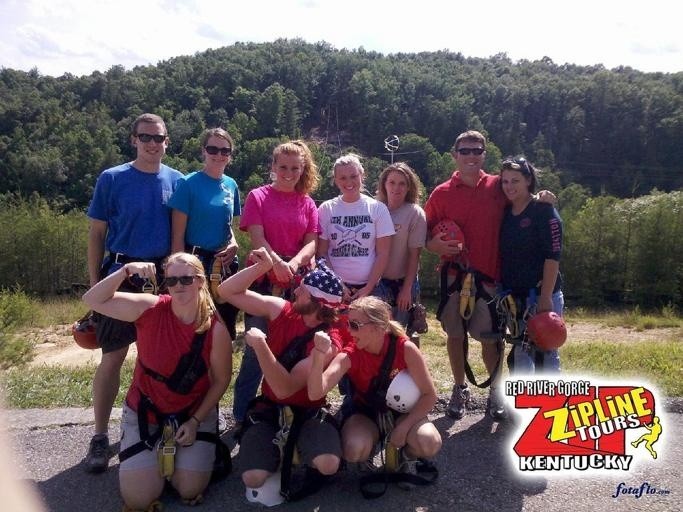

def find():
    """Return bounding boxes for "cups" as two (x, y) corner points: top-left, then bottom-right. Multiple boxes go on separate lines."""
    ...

(165, 276), (198, 287)
(455, 147), (485, 156)
(203, 145), (232, 157)
(133, 134), (167, 144)
(504, 155), (531, 175)
(346, 318), (372, 331)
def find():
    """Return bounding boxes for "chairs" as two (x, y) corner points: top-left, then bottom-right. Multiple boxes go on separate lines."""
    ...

(486, 389), (507, 420)
(84, 434), (109, 475)
(446, 380), (471, 420)
(396, 450), (418, 491)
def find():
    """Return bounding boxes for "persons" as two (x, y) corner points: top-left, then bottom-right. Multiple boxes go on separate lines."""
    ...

(80, 251), (233, 512)
(233, 138), (324, 438)
(218, 245), (343, 489)
(423, 130), (556, 421)
(495, 156), (565, 377)
(317, 154), (397, 397)
(374, 160), (427, 330)
(85, 112), (184, 473)
(165, 126), (244, 343)
(307, 295), (443, 491)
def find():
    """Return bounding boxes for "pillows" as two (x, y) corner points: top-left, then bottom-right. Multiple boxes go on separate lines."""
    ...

(430, 220), (465, 256)
(385, 367), (420, 413)
(526, 309), (568, 352)
(71, 316), (101, 351)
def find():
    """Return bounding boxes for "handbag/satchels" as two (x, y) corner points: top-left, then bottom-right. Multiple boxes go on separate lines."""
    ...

(315, 347), (325, 354)
(123, 265), (131, 279)
(192, 415), (201, 424)
